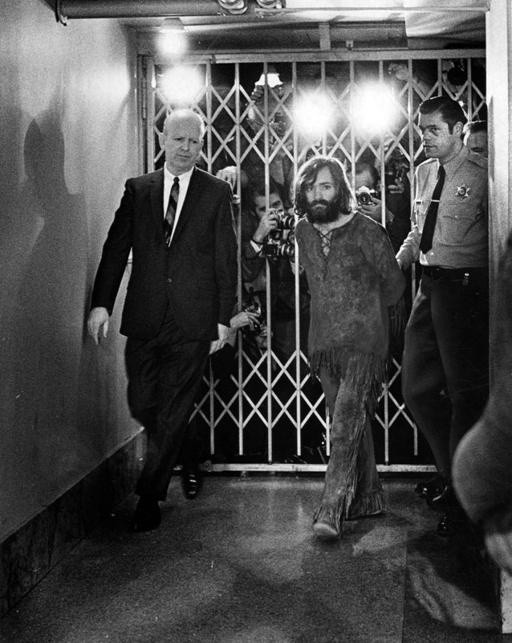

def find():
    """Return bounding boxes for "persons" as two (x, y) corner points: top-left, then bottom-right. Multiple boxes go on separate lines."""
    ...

(217, 63), (488, 364)
(396, 98), (488, 535)
(452, 230), (512, 579)
(294, 156), (406, 537)
(87, 110), (238, 531)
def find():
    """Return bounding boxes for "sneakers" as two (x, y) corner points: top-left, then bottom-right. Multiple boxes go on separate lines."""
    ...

(311, 492), (382, 540)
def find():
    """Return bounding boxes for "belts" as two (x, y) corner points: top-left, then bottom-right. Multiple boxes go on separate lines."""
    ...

(421, 267), (479, 279)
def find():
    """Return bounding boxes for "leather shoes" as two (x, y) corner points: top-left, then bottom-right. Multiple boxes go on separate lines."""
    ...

(135, 494), (161, 531)
(414, 477), (466, 532)
(182, 472), (200, 500)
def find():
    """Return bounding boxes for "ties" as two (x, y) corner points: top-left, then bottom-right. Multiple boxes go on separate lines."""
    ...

(165, 177), (179, 247)
(419, 166), (445, 254)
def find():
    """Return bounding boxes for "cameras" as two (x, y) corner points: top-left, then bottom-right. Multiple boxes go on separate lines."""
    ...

(265, 239), (295, 258)
(355, 190), (376, 209)
(269, 210), (295, 229)
